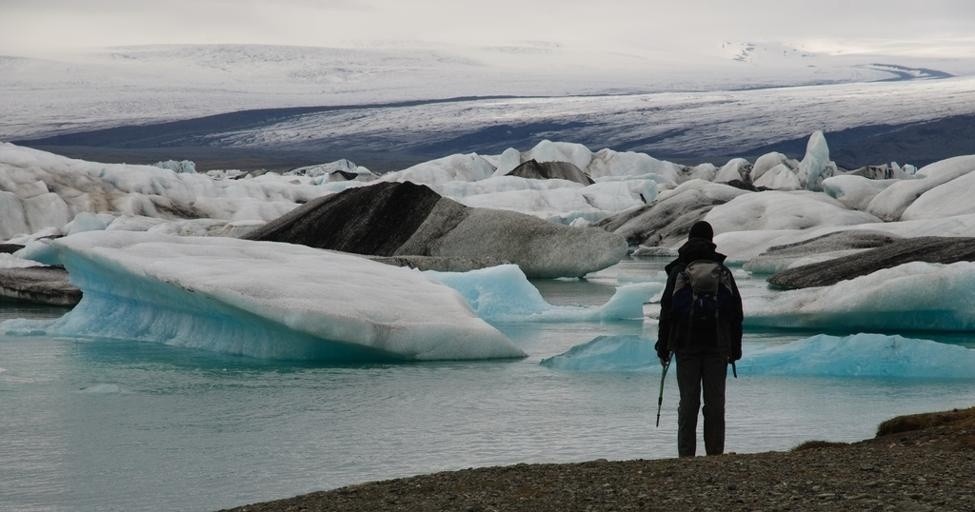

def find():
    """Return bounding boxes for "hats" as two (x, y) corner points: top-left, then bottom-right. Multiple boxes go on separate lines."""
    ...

(689, 221), (713, 242)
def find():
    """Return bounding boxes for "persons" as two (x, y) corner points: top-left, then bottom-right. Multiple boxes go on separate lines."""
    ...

(654, 220), (744, 457)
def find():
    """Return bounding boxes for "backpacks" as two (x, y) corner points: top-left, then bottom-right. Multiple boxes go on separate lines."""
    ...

(670, 259), (733, 357)
